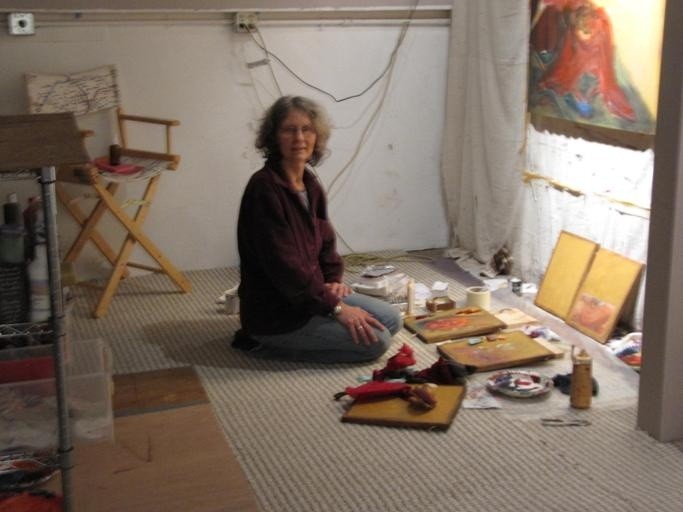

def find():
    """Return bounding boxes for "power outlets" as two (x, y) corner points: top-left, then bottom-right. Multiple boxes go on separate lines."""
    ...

(233, 12), (257, 33)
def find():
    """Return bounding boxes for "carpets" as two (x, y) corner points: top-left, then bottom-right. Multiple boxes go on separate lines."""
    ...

(43, 367), (256, 511)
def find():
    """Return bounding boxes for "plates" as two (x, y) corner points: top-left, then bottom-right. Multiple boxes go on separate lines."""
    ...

(486, 370), (552, 399)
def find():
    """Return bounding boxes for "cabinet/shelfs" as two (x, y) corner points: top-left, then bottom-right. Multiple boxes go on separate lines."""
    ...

(1, 111), (93, 512)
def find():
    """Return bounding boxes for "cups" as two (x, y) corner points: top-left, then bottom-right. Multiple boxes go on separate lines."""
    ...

(223, 288), (239, 314)
(465, 285), (492, 312)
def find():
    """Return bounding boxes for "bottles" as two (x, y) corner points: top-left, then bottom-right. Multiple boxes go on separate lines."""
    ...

(0, 192), (50, 323)
(406, 276), (415, 315)
(571, 350), (595, 410)
(108, 136), (121, 167)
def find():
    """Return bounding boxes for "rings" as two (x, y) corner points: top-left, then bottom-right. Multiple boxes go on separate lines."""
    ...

(357, 325), (362, 330)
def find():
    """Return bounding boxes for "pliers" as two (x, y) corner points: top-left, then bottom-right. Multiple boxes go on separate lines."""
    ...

(541, 418), (590, 426)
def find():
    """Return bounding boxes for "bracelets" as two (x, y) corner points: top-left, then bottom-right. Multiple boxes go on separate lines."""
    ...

(329, 302), (342, 317)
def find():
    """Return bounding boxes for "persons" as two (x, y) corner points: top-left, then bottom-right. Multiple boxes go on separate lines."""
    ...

(230, 95), (402, 364)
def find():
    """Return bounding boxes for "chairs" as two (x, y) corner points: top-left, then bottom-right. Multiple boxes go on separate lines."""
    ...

(21, 65), (191, 318)
